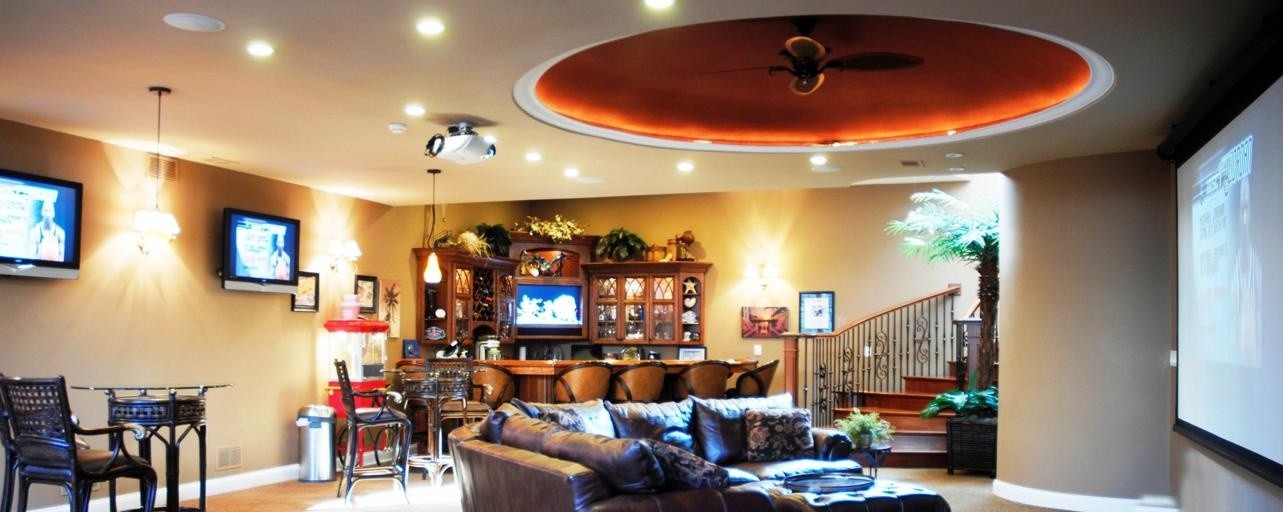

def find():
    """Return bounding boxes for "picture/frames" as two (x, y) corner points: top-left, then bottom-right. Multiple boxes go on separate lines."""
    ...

(677, 346), (707, 360)
(291, 271), (320, 312)
(354, 274), (379, 313)
(799, 291), (836, 334)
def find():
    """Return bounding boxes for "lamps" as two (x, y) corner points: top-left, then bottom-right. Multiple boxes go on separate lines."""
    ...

(116, 87), (181, 264)
(423, 169), (443, 283)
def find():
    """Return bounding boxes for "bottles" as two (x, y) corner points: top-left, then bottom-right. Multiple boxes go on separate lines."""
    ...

(595, 303), (674, 340)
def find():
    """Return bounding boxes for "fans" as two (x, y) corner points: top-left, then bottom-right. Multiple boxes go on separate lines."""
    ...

(686, 19), (927, 95)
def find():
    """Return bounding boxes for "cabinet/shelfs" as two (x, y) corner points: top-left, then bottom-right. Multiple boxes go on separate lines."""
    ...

(504, 231), (602, 341)
(580, 259), (714, 346)
(413, 247), (523, 346)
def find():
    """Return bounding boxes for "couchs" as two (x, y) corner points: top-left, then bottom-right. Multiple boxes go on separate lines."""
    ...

(447, 419), (853, 512)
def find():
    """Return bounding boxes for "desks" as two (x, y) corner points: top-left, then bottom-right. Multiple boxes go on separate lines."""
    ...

(70, 383), (233, 511)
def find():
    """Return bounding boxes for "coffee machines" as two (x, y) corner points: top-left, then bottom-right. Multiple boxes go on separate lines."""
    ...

(474, 339), (502, 360)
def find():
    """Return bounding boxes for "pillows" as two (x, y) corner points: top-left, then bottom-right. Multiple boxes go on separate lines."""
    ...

(781, 407), (816, 460)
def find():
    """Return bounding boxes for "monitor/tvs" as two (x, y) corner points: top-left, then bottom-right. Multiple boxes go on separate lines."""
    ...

(0, 169), (84, 284)
(512, 275), (587, 342)
(219, 204), (301, 295)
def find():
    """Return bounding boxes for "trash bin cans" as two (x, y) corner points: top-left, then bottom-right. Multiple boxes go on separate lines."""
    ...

(295, 404), (337, 483)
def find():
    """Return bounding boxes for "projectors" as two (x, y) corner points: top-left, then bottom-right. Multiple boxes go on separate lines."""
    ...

(420, 125), (503, 172)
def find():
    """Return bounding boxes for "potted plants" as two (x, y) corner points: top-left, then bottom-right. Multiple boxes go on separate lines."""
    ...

(833, 407), (898, 447)
(596, 226), (650, 262)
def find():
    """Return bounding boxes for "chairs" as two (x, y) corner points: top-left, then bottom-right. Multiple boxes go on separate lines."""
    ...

(1, 375), (158, 512)
(0, 374), (90, 512)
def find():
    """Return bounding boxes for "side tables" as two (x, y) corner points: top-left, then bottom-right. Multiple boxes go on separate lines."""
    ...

(844, 443), (891, 481)
